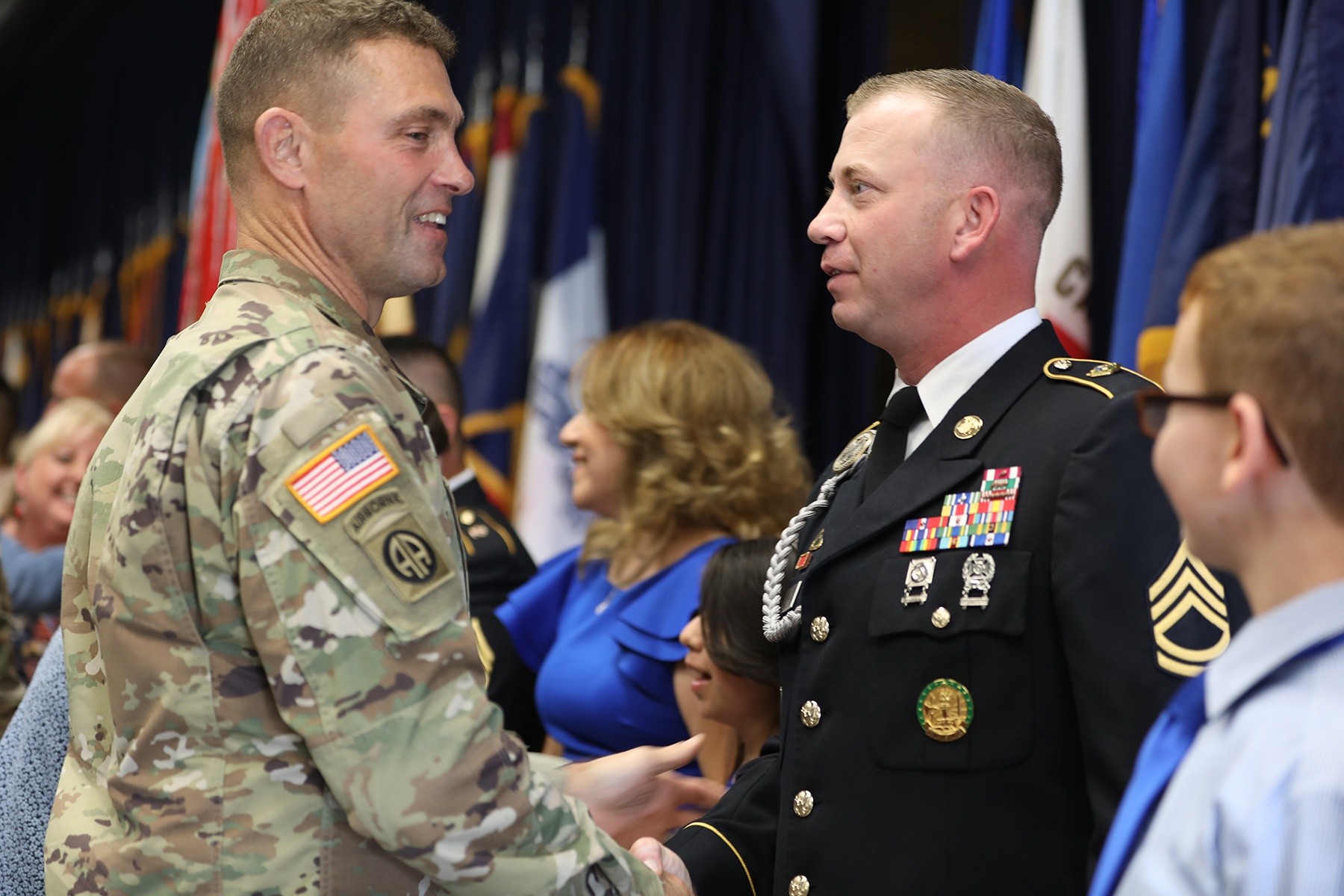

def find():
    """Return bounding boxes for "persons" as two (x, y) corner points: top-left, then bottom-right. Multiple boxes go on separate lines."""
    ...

(2, 324), (147, 896)
(1085, 217), (1344, 896)
(626, 67), (1254, 896)
(493, 319), (814, 844)
(663, 537), (796, 787)
(383, 333), (547, 756)
(44, 0), (729, 896)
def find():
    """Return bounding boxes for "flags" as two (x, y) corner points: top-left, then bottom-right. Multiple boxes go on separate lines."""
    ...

(975, 0), (1344, 376)
(179, 0), (620, 566)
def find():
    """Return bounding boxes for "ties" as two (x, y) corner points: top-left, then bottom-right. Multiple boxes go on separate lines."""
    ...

(1084, 672), (1208, 896)
(853, 384), (927, 510)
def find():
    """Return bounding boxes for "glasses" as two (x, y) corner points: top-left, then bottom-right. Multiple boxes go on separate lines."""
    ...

(1136, 387), (1294, 470)
